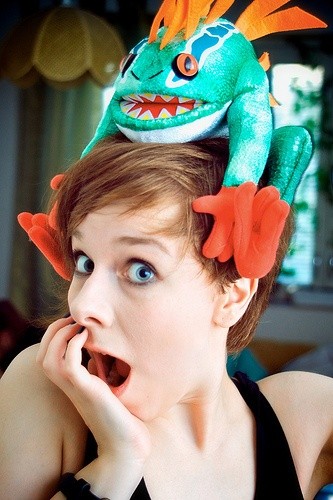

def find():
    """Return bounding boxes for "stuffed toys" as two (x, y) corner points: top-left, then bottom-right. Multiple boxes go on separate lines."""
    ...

(18, 0), (328, 282)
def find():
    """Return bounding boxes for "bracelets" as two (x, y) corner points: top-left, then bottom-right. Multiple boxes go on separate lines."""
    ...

(57, 472), (110, 500)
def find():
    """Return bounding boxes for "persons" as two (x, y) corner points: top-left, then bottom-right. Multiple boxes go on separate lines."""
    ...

(0, 132), (333, 500)
(0, 298), (47, 378)
